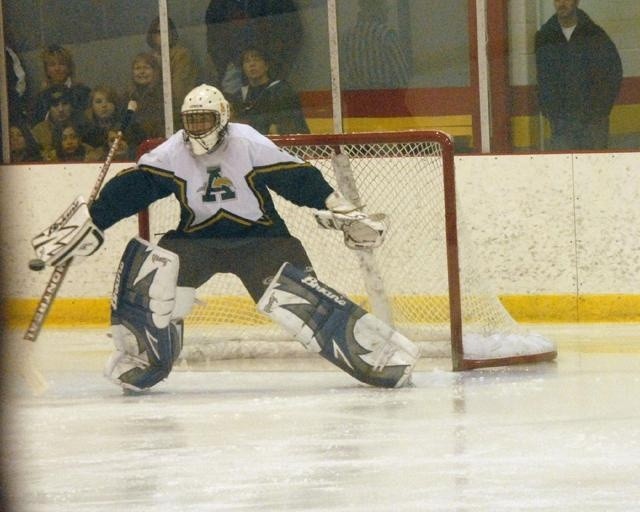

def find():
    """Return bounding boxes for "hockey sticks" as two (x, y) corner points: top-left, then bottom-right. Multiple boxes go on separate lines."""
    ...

(17, 99), (138, 398)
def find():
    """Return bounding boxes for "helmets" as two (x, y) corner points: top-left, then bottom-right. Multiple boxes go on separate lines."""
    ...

(180, 84), (231, 156)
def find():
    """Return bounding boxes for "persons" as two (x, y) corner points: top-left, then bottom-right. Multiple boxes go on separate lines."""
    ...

(340, 0), (412, 117)
(535, 0), (623, 149)
(30, 83), (422, 392)
(6, 0), (311, 164)
